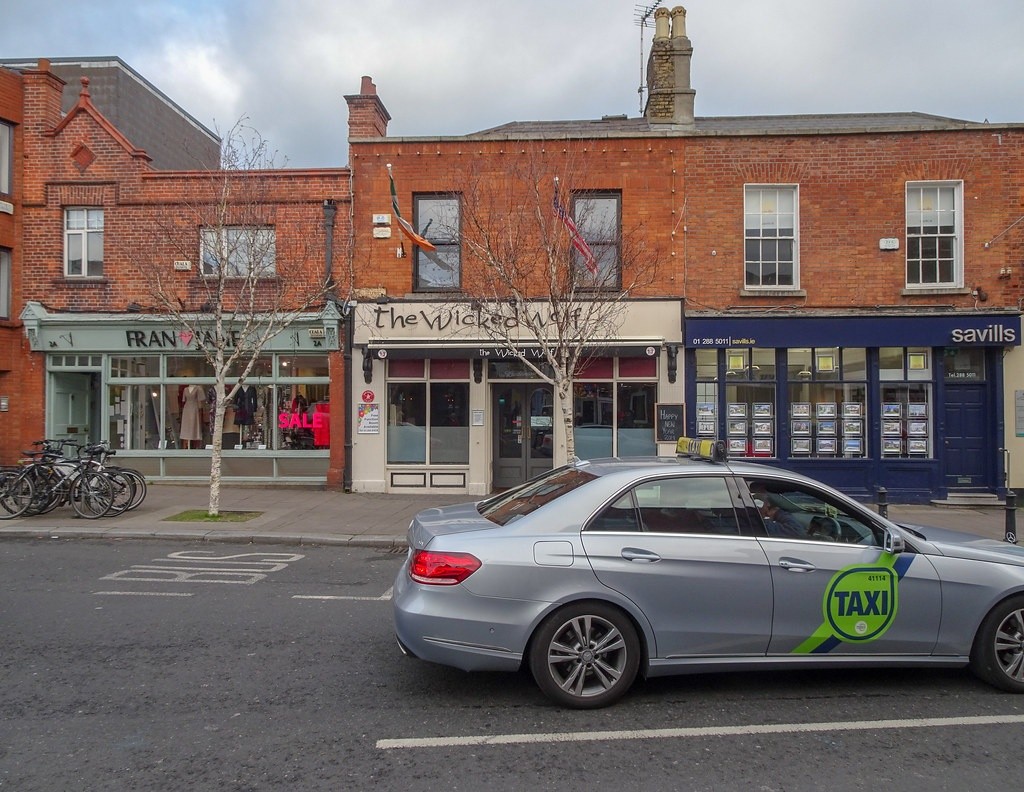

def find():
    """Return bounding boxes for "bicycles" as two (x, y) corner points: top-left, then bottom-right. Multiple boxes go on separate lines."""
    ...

(0, 437), (147, 520)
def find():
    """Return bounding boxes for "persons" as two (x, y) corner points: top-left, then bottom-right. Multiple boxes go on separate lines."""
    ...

(181, 384), (208, 440)
(750, 482), (809, 540)
(291, 393), (308, 413)
(233, 383), (257, 424)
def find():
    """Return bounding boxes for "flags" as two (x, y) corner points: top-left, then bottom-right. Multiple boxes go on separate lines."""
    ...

(389, 173), (435, 252)
(552, 181), (599, 275)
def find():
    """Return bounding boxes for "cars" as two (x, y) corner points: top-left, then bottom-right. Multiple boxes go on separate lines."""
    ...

(391, 436), (1024, 708)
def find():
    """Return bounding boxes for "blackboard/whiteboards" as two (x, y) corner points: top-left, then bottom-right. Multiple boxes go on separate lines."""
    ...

(654, 403), (686, 444)
